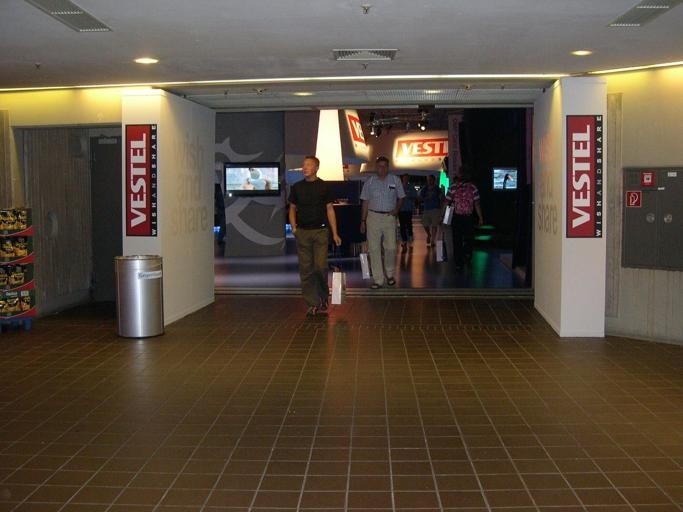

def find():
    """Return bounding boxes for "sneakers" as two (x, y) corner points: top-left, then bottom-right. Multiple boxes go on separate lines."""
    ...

(306, 297), (329, 319)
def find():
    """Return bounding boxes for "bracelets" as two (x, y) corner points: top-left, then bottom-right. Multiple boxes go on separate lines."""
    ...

(360, 219), (366, 223)
(291, 223), (297, 227)
(479, 215), (482, 217)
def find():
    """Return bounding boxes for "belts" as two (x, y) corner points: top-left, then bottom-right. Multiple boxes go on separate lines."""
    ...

(368, 207), (392, 214)
(299, 224), (325, 230)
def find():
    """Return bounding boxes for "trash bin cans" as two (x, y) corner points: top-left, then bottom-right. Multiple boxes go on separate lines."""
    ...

(112, 255), (164, 338)
(471, 225), (501, 288)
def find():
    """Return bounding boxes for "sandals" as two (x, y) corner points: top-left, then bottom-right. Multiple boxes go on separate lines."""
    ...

(387, 276), (395, 286)
(371, 283), (381, 288)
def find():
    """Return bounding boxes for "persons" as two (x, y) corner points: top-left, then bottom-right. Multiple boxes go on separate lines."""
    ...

(287, 156), (342, 317)
(360, 156), (407, 289)
(243, 178), (255, 190)
(416, 174), (446, 248)
(443, 175), (484, 270)
(398, 174), (418, 254)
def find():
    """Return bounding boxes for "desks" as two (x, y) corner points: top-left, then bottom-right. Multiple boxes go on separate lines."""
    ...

(331, 202), (364, 261)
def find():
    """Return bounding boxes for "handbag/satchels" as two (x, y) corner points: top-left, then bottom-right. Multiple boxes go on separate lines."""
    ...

(436, 240), (443, 262)
(359, 252), (370, 280)
(331, 272), (346, 305)
(443, 206), (454, 225)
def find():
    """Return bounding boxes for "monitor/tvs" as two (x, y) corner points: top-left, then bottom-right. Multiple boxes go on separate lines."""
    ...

(492, 166), (518, 191)
(224, 162), (281, 197)
